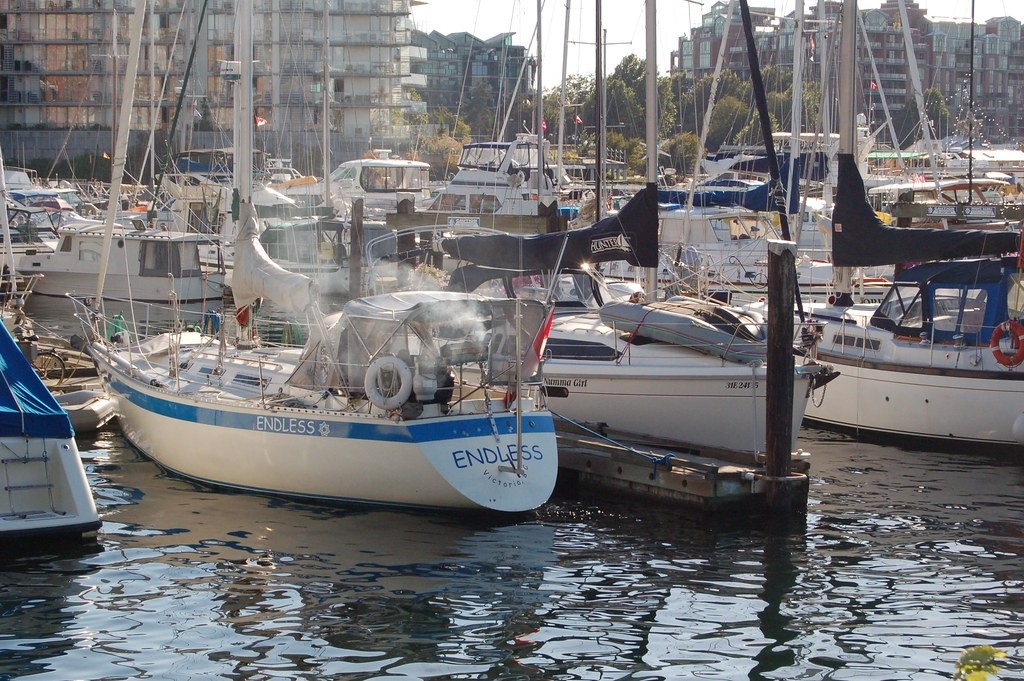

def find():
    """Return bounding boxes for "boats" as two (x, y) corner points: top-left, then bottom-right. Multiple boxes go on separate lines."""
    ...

(0, 0), (1024, 537)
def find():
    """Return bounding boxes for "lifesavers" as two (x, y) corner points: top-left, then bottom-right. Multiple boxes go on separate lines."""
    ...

(12, 220), (18, 227)
(989, 320), (1024, 364)
(85, 205), (96, 217)
(236, 307), (249, 323)
(202, 309), (219, 338)
(364, 355), (412, 410)
